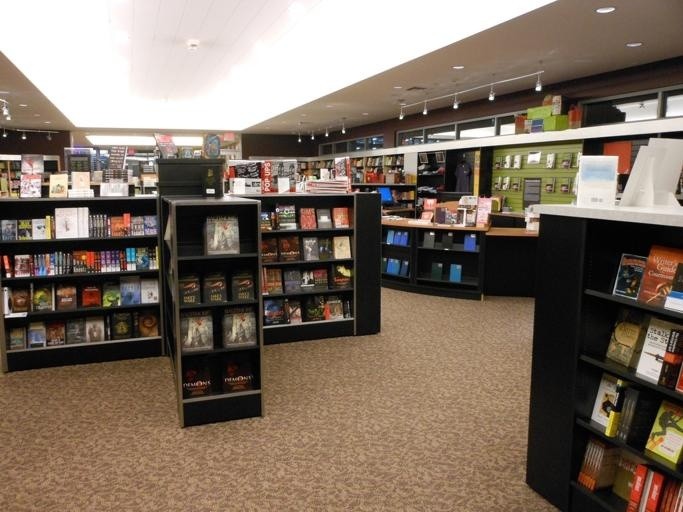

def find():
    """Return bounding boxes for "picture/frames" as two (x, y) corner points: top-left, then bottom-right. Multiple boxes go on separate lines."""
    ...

(418, 152), (428, 163)
(434, 151), (444, 163)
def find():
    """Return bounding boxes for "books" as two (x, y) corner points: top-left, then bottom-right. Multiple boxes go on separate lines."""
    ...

(577, 246), (683, 512)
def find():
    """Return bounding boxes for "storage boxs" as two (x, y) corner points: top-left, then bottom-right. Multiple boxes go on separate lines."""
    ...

(513, 106), (582, 137)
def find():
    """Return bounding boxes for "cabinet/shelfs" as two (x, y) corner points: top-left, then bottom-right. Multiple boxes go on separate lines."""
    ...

(413, 246), (479, 288)
(0, 161), (380, 372)
(572, 286), (683, 511)
(156, 158), (264, 426)
